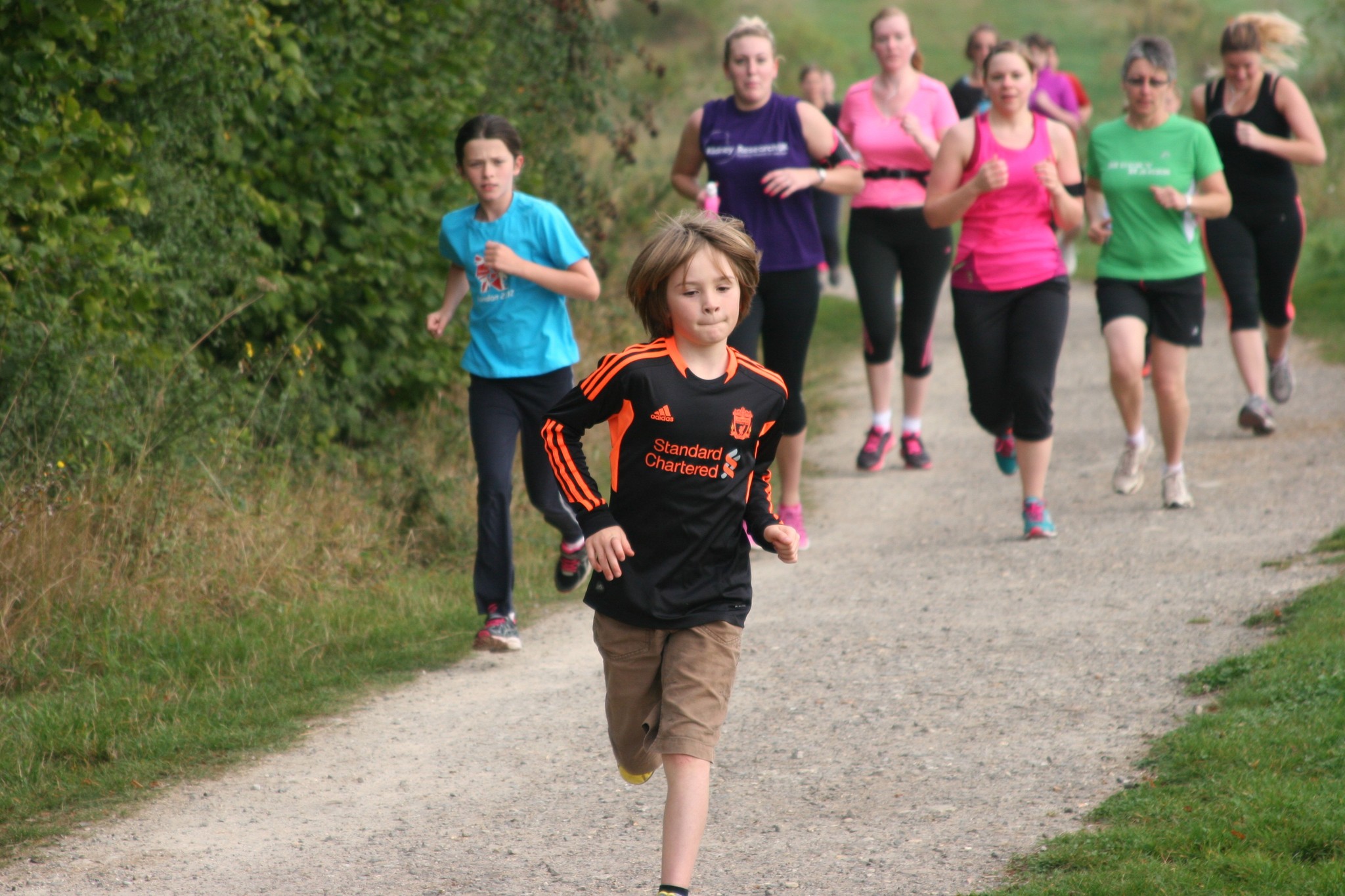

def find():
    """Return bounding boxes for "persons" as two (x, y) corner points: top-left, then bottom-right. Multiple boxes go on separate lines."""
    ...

(426, 115), (602, 654)
(1189, 10), (1327, 439)
(925, 42), (1085, 539)
(539, 209), (808, 896)
(669, 4), (1329, 550)
(835, 6), (960, 473)
(1082, 34), (1231, 510)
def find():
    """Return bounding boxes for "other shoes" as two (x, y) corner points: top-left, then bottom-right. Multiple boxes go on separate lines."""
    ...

(1143, 357), (1156, 378)
(616, 758), (654, 784)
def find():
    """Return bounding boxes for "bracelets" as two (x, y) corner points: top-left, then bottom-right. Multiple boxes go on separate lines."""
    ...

(1186, 194), (1195, 212)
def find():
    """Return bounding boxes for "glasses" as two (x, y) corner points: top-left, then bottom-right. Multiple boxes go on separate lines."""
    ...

(1128, 77), (1167, 89)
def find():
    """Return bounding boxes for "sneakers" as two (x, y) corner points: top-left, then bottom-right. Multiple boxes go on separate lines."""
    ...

(475, 603), (521, 653)
(995, 429), (1019, 475)
(1161, 471), (1191, 507)
(743, 519), (761, 550)
(1112, 425), (1154, 494)
(554, 537), (595, 594)
(1238, 398), (1277, 434)
(898, 432), (931, 469)
(777, 504), (809, 551)
(1022, 498), (1057, 537)
(1268, 346), (1293, 403)
(857, 425), (893, 471)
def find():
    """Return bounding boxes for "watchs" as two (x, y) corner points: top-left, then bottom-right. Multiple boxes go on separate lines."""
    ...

(813, 168), (826, 190)
